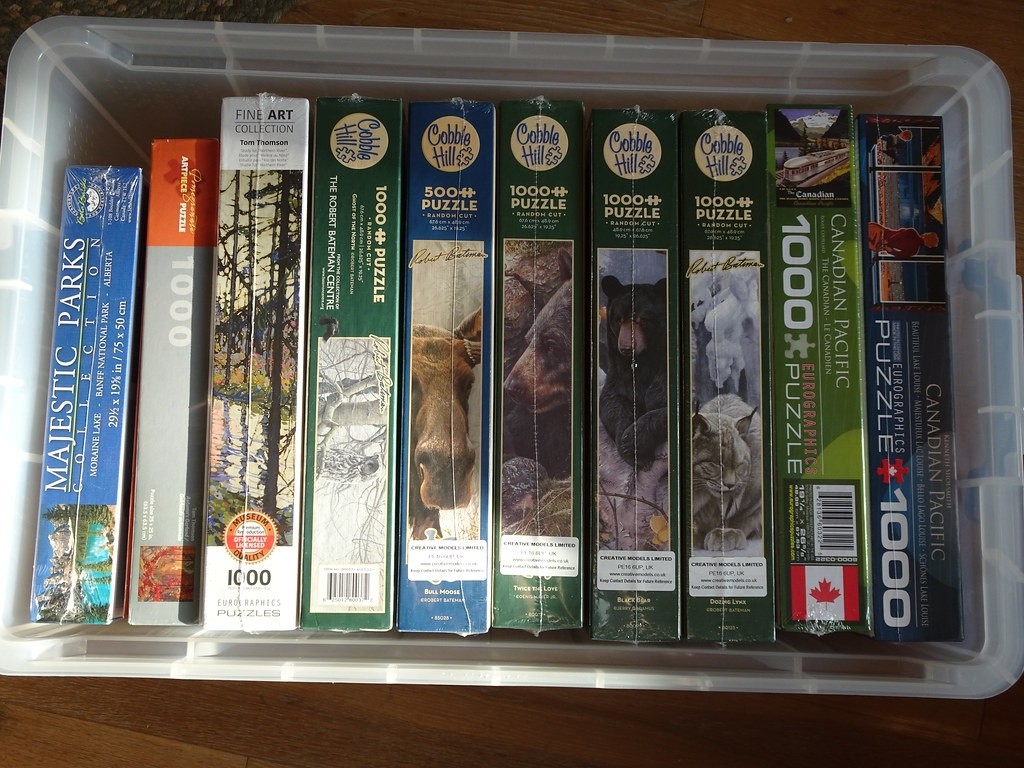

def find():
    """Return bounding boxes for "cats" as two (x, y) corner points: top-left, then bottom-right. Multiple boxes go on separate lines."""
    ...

(693, 394), (765, 550)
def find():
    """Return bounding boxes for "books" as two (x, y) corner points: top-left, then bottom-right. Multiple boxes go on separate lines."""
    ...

(201, 97), (309, 631)
(397, 101), (495, 633)
(29, 168), (149, 625)
(855, 113), (966, 643)
(585, 107), (680, 644)
(299, 97), (402, 629)
(767, 104), (873, 633)
(129, 140), (220, 624)
(491, 99), (584, 629)
(678, 110), (776, 642)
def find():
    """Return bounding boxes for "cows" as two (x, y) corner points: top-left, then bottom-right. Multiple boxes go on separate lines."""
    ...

(410, 308), (481, 541)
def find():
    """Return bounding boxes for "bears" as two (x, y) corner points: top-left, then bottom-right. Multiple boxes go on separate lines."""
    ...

(600, 272), (669, 472)
(500, 246), (572, 478)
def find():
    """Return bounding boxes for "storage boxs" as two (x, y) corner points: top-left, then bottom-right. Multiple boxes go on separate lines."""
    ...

(0, 16), (1024, 703)
